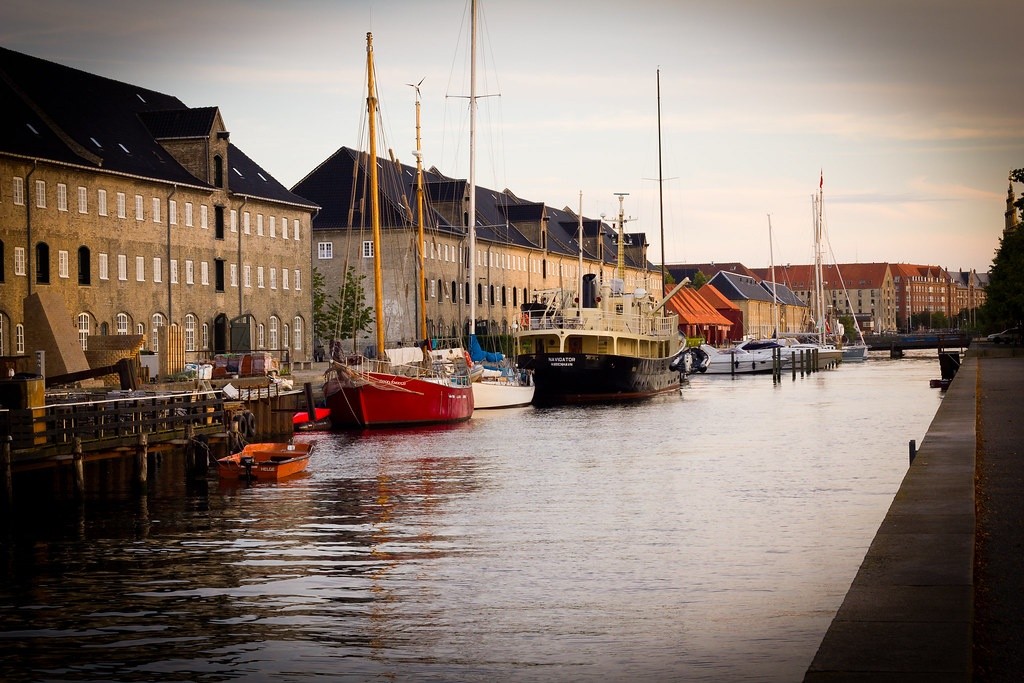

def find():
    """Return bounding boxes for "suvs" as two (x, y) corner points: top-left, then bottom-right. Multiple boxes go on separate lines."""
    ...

(987, 327), (1024, 345)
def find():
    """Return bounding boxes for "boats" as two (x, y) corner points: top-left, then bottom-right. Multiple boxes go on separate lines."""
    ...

(518, 187), (687, 408)
(690, 341), (790, 378)
(216, 440), (317, 486)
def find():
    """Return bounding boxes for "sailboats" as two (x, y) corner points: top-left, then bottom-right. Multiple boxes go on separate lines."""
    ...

(806, 173), (871, 361)
(322, 30), (474, 429)
(762, 214), (843, 374)
(431, 1), (534, 412)
